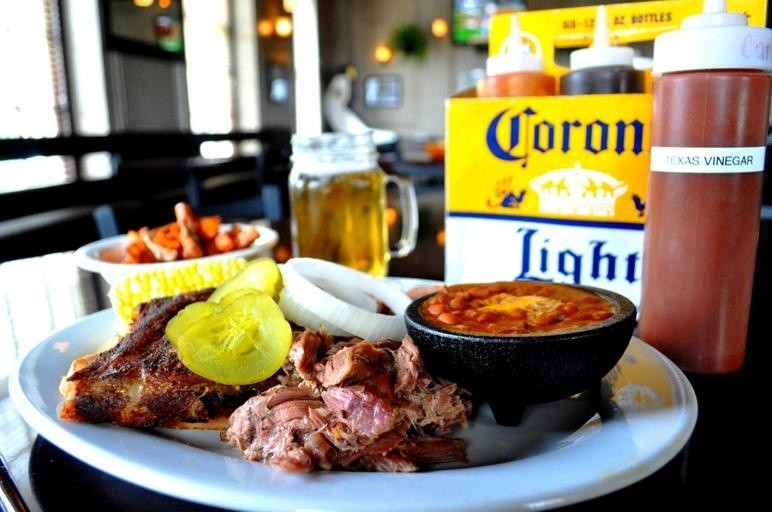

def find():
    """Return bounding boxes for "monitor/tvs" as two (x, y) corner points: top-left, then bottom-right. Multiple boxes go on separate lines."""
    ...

(448, 1), (526, 46)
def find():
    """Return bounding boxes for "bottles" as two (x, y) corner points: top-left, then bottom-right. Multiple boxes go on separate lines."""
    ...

(636, 0), (772, 378)
(473, 15), (556, 98)
(557, 4), (635, 97)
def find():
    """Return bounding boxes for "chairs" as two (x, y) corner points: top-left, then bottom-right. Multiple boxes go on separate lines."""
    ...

(1, 139), (444, 281)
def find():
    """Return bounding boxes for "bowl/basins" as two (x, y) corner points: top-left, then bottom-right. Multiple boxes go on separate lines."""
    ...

(69, 219), (283, 293)
(398, 277), (637, 426)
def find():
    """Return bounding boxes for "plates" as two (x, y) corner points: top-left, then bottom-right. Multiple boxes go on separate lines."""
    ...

(6, 276), (701, 512)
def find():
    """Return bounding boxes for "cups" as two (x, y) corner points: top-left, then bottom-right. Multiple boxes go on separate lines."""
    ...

(285, 126), (421, 278)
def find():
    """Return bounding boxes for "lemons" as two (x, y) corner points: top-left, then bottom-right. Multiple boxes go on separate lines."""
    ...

(164, 257), (293, 386)
